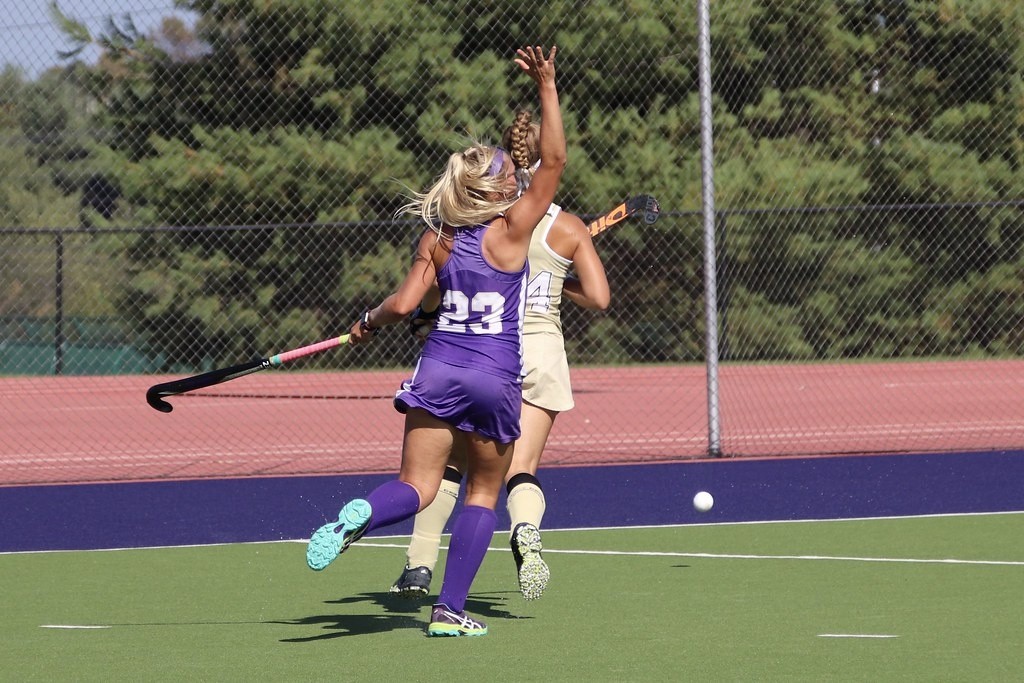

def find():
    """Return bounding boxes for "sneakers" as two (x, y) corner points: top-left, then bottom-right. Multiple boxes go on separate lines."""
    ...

(389, 563), (433, 598)
(307, 498), (373, 571)
(427, 604), (489, 638)
(510, 523), (550, 601)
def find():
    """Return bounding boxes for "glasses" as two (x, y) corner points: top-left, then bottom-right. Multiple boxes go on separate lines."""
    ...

(501, 170), (520, 184)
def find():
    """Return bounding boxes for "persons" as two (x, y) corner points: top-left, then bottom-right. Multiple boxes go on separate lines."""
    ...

(306, 45), (568, 636)
(390, 109), (610, 601)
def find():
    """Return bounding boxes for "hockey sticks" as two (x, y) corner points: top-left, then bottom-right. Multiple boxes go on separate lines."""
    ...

(415, 191), (662, 341)
(145, 326), (383, 414)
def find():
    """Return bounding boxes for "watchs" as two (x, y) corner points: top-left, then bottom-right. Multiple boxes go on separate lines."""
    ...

(360, 310), (375, 331)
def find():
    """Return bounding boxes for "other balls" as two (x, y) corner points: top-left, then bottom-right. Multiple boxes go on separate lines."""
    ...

(692, 490), (715, 513)
